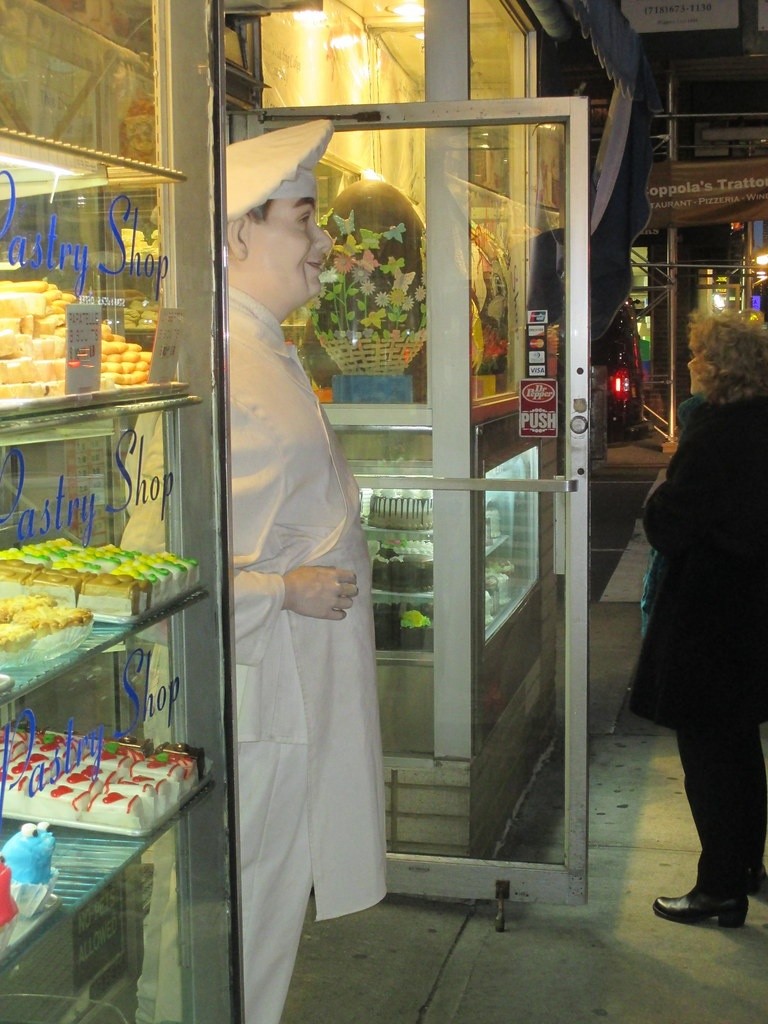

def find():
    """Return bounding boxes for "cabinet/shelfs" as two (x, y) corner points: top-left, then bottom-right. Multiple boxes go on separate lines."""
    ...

(0, 5), (243, 1024)
(328, 410), (545, 751)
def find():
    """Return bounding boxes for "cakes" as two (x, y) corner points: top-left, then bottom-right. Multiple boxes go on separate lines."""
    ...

(366, 489), (435, 653)
(485, 506), (513, 628)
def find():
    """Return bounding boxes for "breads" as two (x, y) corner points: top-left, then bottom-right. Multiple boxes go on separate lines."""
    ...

(0, 279), (159, 401)
(119, 228), (159, 262)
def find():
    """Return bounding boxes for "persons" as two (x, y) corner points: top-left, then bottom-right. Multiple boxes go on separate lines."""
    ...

(630, 315), (768, 925)
(133, 118), (391, 1023)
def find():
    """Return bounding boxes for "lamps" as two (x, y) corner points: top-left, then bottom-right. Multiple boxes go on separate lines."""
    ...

(329, 16), (362, 54)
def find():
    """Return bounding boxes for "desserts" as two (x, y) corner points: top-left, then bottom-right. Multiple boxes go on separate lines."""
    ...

(0, 726), (207, 833)
(0, 537), (202, 670)
(0, 821), (61, 953)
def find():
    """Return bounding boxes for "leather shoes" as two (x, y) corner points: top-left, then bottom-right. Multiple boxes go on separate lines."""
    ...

(747, 863), (765, 892)
(653, 876), (748, 927)
